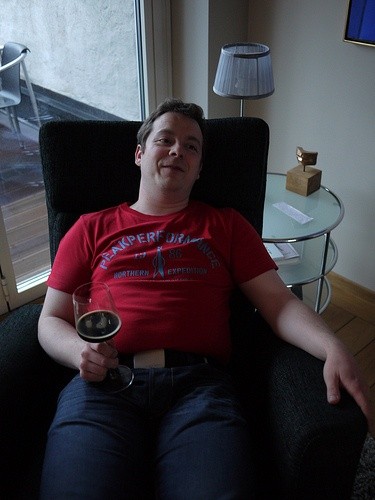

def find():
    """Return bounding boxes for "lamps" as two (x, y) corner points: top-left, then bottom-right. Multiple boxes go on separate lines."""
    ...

(213, 42), (276, 117)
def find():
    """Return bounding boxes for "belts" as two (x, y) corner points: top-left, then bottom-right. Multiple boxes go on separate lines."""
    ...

(114, 348), (205, 369)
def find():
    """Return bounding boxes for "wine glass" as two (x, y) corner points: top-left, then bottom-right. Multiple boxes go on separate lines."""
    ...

(73, 281), (135, 394)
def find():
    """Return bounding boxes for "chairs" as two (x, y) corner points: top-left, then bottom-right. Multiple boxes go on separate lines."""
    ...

(0, 115), (366, 500)
(0, 42), (42, 149)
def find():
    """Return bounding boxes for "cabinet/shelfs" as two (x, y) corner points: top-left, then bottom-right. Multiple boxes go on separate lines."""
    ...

(262, 172), (343, 315)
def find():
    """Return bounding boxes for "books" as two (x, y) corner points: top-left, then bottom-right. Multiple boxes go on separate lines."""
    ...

(261, 241), (300, 261)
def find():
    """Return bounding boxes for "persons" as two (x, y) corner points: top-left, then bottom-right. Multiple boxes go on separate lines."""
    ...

(36, 100), (375, 500)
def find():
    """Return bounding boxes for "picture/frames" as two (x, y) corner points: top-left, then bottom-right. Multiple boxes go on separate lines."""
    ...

(341, 0), (375, 48)
